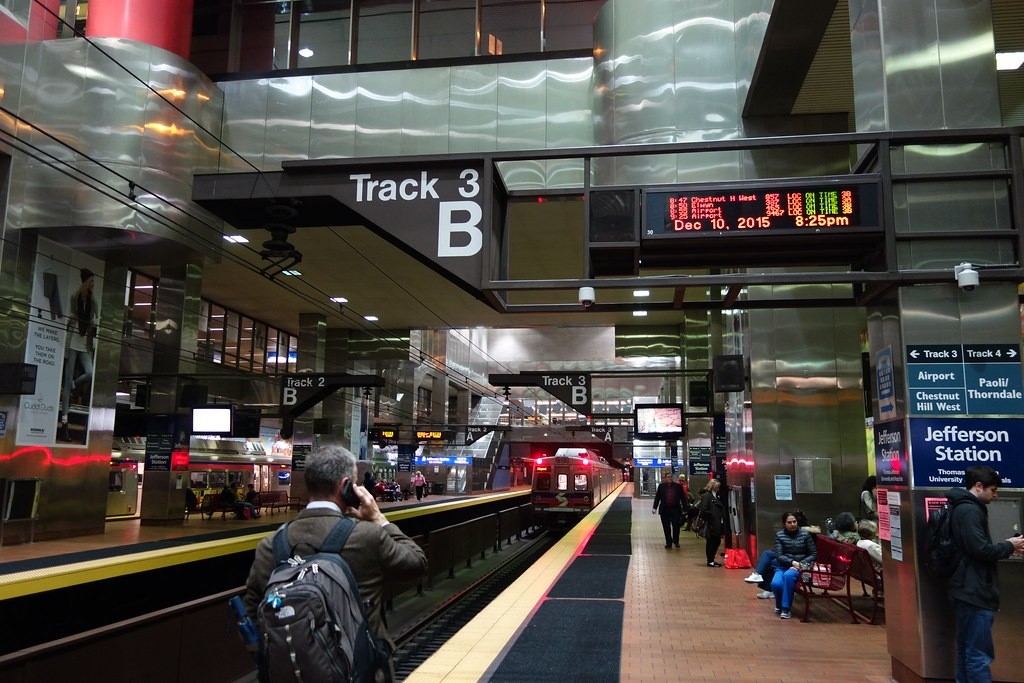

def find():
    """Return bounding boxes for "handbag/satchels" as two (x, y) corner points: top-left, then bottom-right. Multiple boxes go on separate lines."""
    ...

(810, 561), (832, 588)
(691, 514), (711, 538)
(724, 546), (752, 569)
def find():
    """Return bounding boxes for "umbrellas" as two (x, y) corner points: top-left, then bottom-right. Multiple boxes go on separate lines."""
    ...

(228, 596), (261, 662)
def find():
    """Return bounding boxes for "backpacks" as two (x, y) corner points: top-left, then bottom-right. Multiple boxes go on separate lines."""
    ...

(256, 520), (378, 683)
(924, 498), (975, 584)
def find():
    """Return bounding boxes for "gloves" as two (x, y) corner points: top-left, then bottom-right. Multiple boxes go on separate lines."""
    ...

(652, 509), (657, 513)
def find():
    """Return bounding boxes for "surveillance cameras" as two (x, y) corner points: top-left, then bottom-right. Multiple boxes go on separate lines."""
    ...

(579, 287), (595, 307)
(958, 270), (980, 291)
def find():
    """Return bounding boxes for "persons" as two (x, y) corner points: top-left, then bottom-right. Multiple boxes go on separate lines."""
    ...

(376, 478), (413, 502)
(676, 474), (703, 531)
(698, 478), (726, 567)
(944, 465), (1024, 683)
(412, 470), (427, 501)
(858, 475), (878, 518)
(743, 510), (821, 619)
(218, 483), (261, 520)
(653, 473), (687, 549)
(235, 445), (426, 683)
(825, 511), (883, 587)
(185, 488), (198, 508)
(362, 472), (376, 492)
(59, 268), (98, 442)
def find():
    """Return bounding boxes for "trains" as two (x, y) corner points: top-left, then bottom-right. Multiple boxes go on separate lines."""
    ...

(530, 447), (624, 538)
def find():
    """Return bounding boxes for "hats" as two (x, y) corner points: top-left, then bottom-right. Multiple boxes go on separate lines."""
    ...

(80, 267), (95, 282)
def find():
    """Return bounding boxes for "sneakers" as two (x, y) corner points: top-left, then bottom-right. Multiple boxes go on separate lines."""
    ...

(775, 607), (781, 613)
(745, 573), (764, 583)
(757, 590), (775, 598)
(780, 609), (792, 618)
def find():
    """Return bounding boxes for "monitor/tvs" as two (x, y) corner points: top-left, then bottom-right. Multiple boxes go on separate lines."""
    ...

(191, 404), (233, 436)
(634, 403), (684, 441)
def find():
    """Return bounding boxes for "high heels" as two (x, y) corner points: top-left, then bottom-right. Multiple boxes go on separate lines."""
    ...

(682, 528), (690, 531)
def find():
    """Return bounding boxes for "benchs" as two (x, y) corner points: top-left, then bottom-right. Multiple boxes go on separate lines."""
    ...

(186, 494), (208, 520)
(210, 495), (240, 521)
(257, 490), (305, 515)
(247, 491), (259, 514)
(794, 533), (885, 625)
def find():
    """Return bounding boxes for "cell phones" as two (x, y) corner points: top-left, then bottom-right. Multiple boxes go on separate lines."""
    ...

(341, 480), (359, 510)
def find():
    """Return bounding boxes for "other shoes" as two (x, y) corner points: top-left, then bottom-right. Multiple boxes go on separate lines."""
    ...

(707, 561), (722, 567)
(673, 541), (680, 548)
(665, 543), (672, 548)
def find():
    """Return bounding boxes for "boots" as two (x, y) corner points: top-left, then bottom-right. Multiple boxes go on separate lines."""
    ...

(60, 423), (72, 442)
